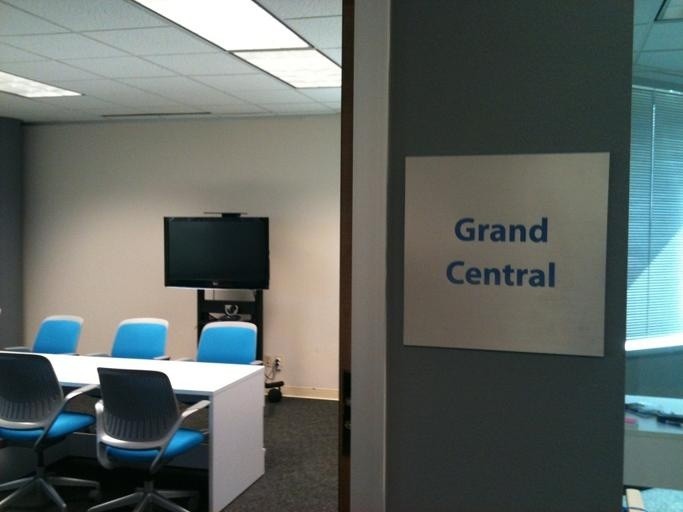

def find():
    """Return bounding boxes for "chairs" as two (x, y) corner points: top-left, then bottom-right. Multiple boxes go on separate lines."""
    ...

(173, 321), (265, 408)
(0, 350), (103, 512)
(84, 317), (171, 399)
(3, 314), (83, 356)
(89, 366), (211, 512)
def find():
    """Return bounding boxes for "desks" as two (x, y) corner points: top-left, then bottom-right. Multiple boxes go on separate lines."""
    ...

(1, 351), (264, 512)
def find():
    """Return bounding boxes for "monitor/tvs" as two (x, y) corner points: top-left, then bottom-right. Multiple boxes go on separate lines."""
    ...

(163, 216), (270, 292)
